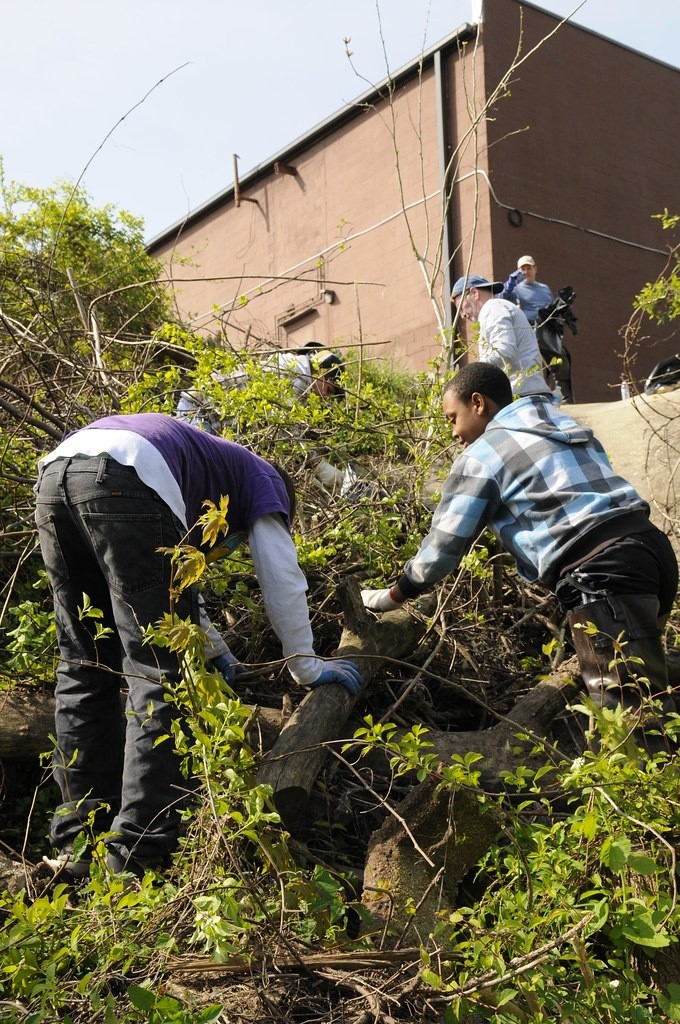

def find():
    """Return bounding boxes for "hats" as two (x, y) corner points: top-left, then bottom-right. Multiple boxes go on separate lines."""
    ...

(311, 350), (345, 402)
(518, 255), (536, 268)
(452, 275), (504, 296)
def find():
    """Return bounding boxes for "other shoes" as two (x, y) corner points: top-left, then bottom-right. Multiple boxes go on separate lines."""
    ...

(59, 844), (94, 872)
(107, 852), (163, 885)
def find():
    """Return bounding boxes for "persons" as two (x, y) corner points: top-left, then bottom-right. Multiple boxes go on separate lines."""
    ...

(361, 362), (680, 772)
(452, 255), (573, 405)
(34, 413), (360, 886)
(177, 343), (345, 489)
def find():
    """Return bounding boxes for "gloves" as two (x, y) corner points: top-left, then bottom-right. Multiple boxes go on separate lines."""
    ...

(211, 653), (249, 680)
(307, 658), (363, 696)
(360, 586), (403, 611)
(504, 272), (522, 292)
(315, 460), (345, 487)
